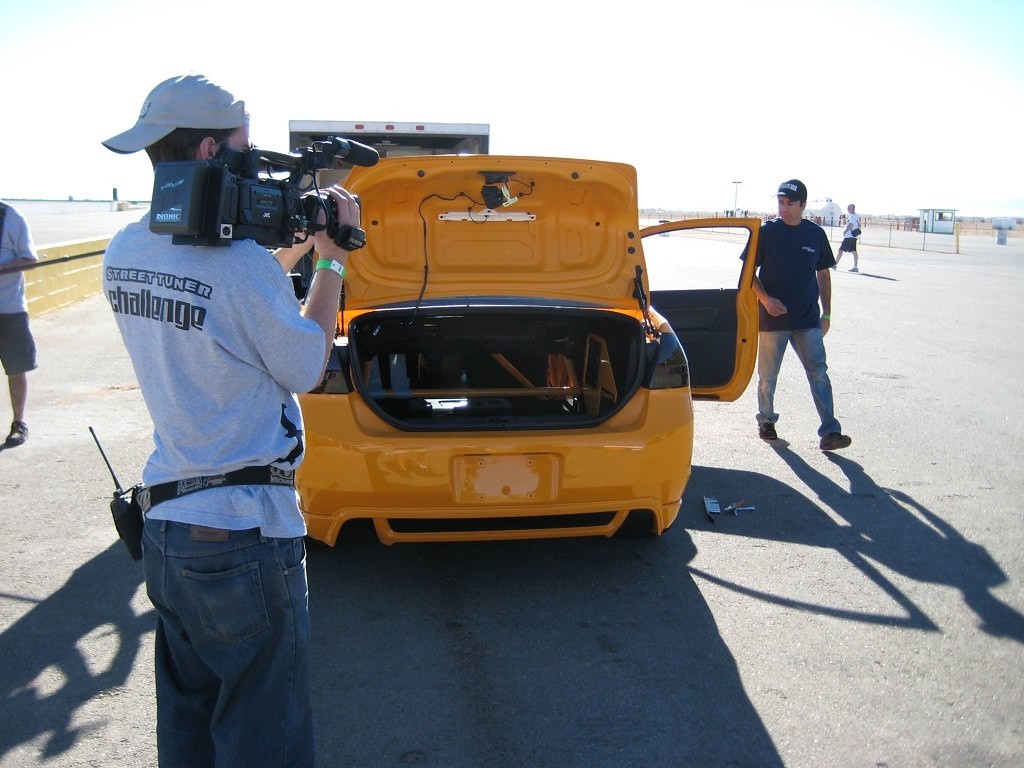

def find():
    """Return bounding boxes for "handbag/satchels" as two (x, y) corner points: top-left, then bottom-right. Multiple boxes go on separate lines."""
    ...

(851, 228), (861, 237)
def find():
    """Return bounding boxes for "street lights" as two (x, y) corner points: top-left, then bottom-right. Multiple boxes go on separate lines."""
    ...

(732, 182), (742, 210)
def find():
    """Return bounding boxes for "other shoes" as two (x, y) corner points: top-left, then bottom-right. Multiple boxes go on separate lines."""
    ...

(848, 268), (858, 272)
(820, 433), (851, 450)
(831, 265), (837, 270)
(759, 423), (777, 440)
(7, 421), (28, 445)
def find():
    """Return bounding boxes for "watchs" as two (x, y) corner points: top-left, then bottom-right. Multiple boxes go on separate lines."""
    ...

(315, 259), (345, 279)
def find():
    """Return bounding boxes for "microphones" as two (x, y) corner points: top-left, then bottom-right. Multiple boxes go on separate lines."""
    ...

(324, 135), (380, 167)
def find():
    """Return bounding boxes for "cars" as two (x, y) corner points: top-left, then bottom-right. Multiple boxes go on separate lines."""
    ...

(290, 152), (763, 546)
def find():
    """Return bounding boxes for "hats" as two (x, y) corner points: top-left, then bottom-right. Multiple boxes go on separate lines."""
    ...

(777, 180), (807, 201)
(102, 76), (249, 153)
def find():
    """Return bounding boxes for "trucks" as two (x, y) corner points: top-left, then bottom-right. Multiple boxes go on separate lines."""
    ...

(287, 119), (492, 296)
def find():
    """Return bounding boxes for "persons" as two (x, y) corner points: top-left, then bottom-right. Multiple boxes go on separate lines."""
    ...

(829, 204), (859, 272)
(99, 72), (360, 768)
(0, 201), (40, 445)
(739, 179), (852, 451)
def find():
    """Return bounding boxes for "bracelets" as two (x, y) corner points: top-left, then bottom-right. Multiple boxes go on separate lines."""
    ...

(821, 314), (830, 319)
